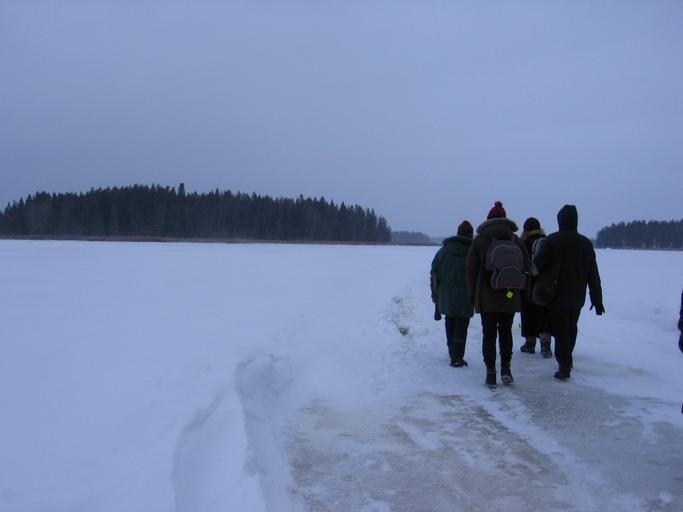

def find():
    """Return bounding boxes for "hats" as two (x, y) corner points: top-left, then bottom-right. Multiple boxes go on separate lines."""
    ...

(457, 220), (473, 238)
(523, 218), (540, 231)
(487, 201), (506, 219)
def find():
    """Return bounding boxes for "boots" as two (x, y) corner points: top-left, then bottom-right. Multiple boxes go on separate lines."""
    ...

(555, 355), (572, 380)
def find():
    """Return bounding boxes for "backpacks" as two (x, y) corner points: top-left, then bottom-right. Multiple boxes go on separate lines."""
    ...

(524, 230), (547, 276)
(484, 235), (527, 290)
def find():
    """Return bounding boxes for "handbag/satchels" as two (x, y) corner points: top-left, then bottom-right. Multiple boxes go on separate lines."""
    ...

(531, 261), (560, 306)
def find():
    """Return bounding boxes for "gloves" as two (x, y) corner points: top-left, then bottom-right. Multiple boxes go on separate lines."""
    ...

(590, 301), (605, 315)
(431, 294), (438, 303)
(469, 295), (475, 305)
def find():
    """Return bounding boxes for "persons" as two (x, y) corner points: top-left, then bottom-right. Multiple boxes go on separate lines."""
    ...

(533, 205), (606, 378)
(521, 218), (553, 358)
(678, 291), (683, 415)
(466, 201), (531, 389)
(430, 220), (480, 368)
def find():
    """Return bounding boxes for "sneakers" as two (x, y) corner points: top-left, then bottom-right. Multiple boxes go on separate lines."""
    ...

(501, 367), (513, 384)
(486, 368), (496, 387)
(450, 355), (467, 367)
(521, 342), (536, 353)
(541, 342), (552, 358)
